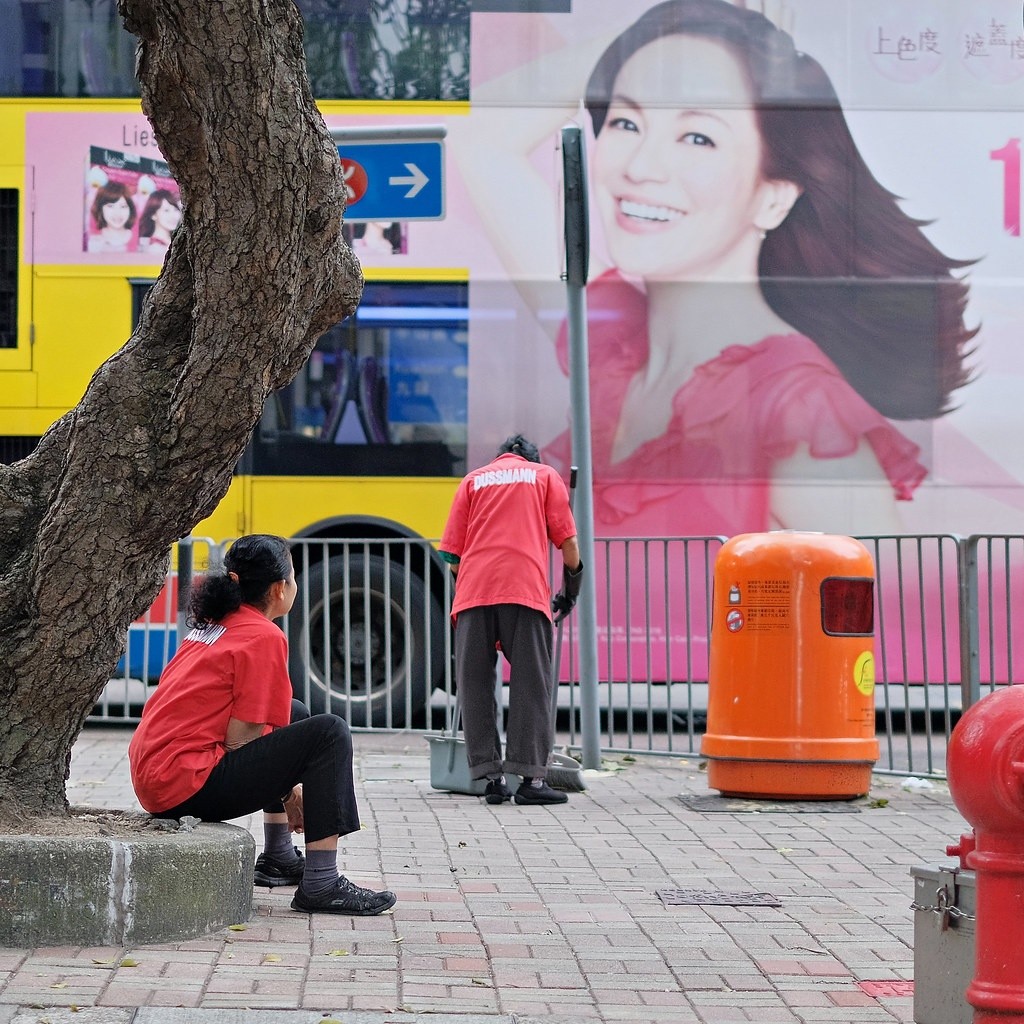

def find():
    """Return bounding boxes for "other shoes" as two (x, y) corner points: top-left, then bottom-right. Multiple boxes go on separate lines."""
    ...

(515, 780), (568, 805)
(485, 778), (512, 805)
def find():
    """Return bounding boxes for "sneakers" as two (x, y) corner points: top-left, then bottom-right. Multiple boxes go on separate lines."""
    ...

(290, 875), (397, 915)
(254, 846), (305, 885)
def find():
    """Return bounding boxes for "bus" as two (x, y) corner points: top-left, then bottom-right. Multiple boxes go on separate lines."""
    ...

(0, 98), (469, 729)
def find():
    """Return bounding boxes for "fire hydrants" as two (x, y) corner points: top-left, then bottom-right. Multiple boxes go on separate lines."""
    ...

(946, 685), (1024, 1024)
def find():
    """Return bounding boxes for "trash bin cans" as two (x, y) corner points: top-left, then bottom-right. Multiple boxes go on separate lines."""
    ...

(701, 530), (880, 800)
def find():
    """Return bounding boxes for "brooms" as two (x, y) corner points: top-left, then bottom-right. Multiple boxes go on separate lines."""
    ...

(540, 465), (586, 795)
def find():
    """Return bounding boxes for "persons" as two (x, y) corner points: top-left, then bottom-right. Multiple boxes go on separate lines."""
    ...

(85, 165), (181, 253)
(128, 534), (397, 915)
(438, 436), (584, 806)
(444, 0), (1023, 538)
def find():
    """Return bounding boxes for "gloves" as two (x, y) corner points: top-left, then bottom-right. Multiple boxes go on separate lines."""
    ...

(553, 561), (584, 622)
(451, 570), (457, 583)
